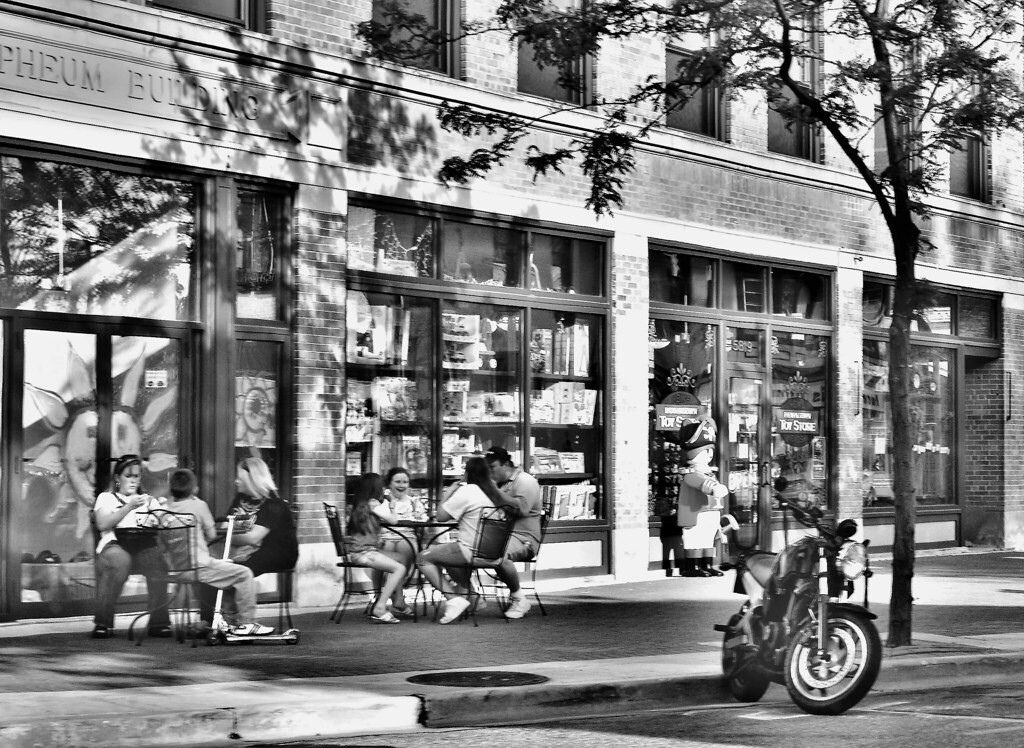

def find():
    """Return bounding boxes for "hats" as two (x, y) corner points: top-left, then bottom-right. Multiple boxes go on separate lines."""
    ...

(484, 446), (508, 464)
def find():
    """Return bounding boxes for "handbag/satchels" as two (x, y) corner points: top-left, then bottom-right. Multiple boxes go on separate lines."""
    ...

(210, 512), (263, 563)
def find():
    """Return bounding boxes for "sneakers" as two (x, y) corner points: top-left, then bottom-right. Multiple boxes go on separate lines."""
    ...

(468, 594), (488, 611)
(232, 622), (276, 636)
(439, 596), (472, 624)
(505, 597), (531, 619)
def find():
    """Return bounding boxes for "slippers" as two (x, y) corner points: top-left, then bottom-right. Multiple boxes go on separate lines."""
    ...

(368, 611), (401, 624)
(389, 606), (414, 616)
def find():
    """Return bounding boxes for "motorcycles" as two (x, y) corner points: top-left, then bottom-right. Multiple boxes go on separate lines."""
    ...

(714, 476), (884, 716)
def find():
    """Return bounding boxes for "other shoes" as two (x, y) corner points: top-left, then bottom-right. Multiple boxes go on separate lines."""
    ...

(94, 623), (108, 639)
(149, 627), (174, 638)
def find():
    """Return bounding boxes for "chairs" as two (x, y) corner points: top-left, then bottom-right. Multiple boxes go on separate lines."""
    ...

(322, 501), (553, 628)
(86, 504), (300, 643)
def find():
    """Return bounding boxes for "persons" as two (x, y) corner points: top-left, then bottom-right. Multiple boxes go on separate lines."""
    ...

(161, 468), (275, 636)
(678, 415), (729, 578)
(378, 466), (429, 586)
(91, 452), (174, 638)
(443, 446), (543, 619)
(346, 472), (415, 624)
(182, 456), (299, 636)
(416, 456), (511, 625)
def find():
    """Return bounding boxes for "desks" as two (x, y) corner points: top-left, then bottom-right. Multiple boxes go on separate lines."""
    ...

(129, 525), (252, 645)
(381, 519), (459, 623)
